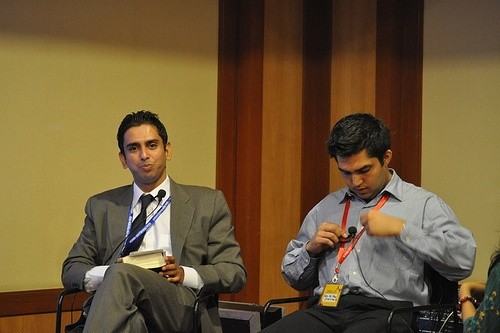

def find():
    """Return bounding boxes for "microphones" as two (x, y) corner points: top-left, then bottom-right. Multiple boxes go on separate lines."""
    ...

(348, 226), (358, 239)
(157, 189), (166, 203)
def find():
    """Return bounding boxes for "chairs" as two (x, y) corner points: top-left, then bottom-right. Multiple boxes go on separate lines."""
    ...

(56, 288), (219, 333)
(262, 263), (461, 333)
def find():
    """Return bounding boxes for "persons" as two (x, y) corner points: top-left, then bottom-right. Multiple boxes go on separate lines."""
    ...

(60, 109), (247, 333)
(457, 244), (500, 333)
(258, 113), (476, 333)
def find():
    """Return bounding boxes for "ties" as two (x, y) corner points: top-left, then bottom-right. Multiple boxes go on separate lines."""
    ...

(121, 194), (155, 257)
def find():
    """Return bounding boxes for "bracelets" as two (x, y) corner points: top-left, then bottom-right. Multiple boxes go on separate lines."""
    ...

(458, 295), (477, 311)
